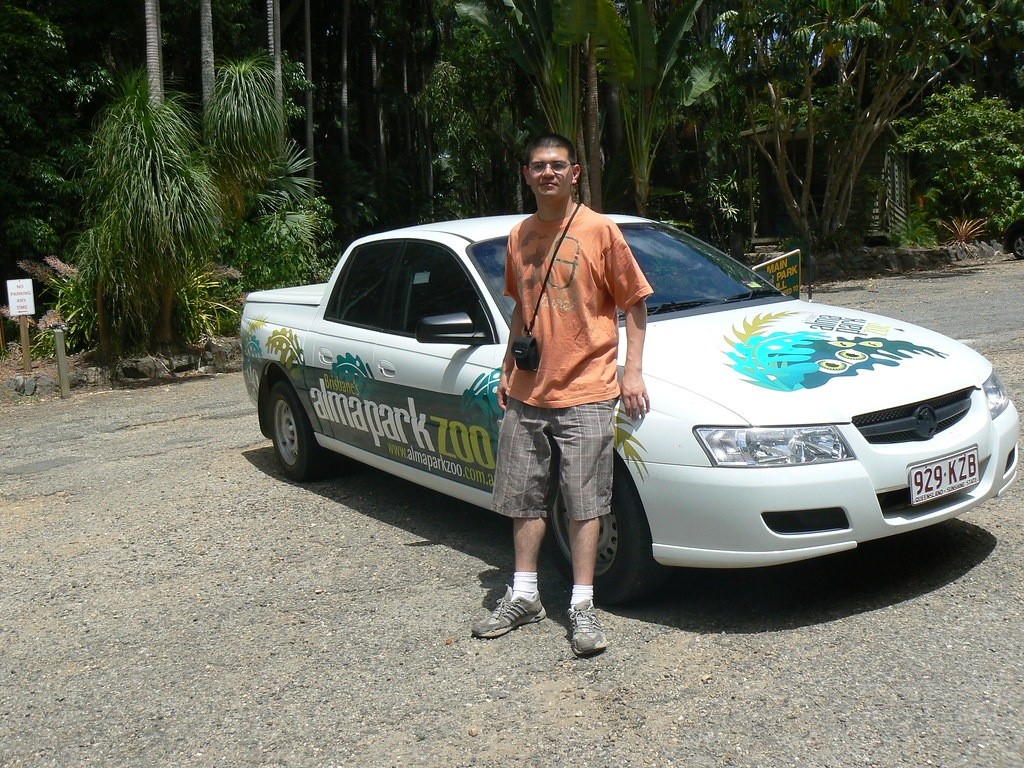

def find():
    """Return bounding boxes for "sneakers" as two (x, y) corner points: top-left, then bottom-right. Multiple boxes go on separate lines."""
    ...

(566, 599), (607, 654)
(471, 583), (546, 638)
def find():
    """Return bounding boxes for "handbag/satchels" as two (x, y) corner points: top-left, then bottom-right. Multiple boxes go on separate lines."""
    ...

(511, 334), (539, 372)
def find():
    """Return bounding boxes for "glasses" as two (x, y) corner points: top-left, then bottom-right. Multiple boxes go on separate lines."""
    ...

(527, 161), (576, 175)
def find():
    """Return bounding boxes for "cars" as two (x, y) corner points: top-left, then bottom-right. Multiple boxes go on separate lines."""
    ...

(239, 212), (1024, 610)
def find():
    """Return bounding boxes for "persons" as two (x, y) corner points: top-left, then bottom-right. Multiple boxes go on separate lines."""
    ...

(472, 133), (654, 656)
